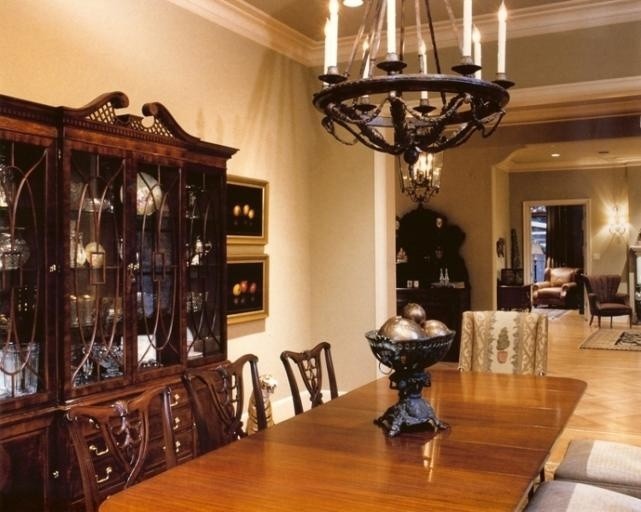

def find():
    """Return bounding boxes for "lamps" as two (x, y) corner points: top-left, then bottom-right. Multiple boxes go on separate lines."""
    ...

(531, 243), (544, 307)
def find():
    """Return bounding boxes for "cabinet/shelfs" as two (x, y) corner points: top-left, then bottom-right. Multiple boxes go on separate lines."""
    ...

(397, 287), (470, 362)
(60, 90), (240, 509)
(499, 284), (532, 312)
(1, 94), (62, 512)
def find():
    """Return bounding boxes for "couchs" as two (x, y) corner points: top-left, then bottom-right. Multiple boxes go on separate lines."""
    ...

(533, 267), (583, 308)
(590, 274), (629, 305)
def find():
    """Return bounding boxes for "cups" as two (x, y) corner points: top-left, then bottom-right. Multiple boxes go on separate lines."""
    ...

(1, 343), (41, 395)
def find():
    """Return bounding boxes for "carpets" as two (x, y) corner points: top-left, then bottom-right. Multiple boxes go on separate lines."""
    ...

(532, 307), (576, 321)
(580, 327), (641, 351)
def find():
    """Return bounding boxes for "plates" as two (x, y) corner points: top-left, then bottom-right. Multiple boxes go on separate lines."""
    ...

(118, 172), (164, 216)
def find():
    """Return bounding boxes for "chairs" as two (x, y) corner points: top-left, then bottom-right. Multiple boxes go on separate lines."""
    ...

(579, 273), (634, 329)
(66, 384), (177, 511)
(181, 354), (268, 454)
(457, 310), (550, 377)
(280, 342), (339, 416)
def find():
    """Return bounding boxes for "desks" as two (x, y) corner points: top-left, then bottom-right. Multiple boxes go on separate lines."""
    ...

(99, 368), (587, 512)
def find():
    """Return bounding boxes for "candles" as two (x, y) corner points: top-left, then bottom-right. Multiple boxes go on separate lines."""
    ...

(324, 17), (332, 86)
(362, 36), (370, 97)
(472, 25), (483, 80)
(462, 1), (475, 57)
(387, 0), (396, 55)
(497, 1), (507, 76)
(328, 0), (339, 68)
(421, 39), (428, 99)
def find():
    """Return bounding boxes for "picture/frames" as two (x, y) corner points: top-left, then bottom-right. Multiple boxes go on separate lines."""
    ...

(226, 174), (269, 244)
(226, 255), (270, 324)
